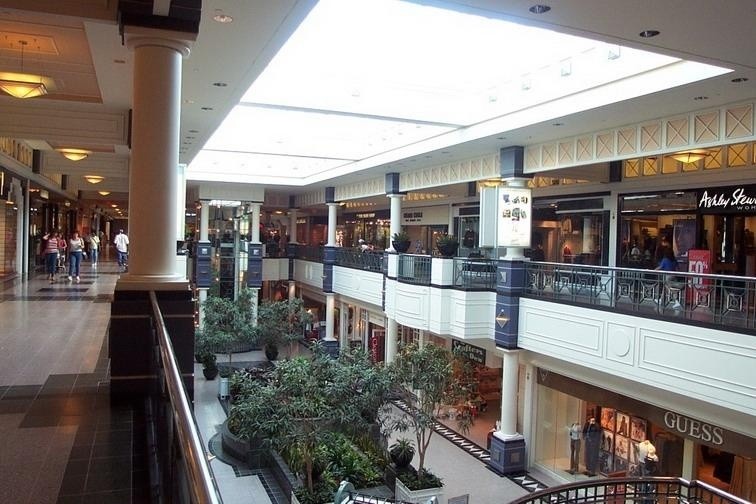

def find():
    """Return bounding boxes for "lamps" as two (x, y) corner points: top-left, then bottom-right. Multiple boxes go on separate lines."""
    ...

(99, 191), (125, 216)
(61, 149), (89, 161)
(84, 174), (103, 184)
(0, 78), (48, 100)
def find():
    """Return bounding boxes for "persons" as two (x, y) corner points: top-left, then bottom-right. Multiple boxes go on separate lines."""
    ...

(35, 227), (105, 283)
(674, 220), (695, 256)
(565, 407), (657, 503)
(185, 232), (193, 240)
(226, 230), (231, 238)
(318, 239), (324, 263)
(654, 247), (682, 308)
(303, 309), (314, 341)
(358, 238), (380, 271)
(208, 233), (215, 243)
(113, 229), (129, 266)
(530, 238), (545, 291)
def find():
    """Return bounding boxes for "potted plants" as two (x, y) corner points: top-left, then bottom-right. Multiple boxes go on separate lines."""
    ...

(389, 436), (415, 467)
(436, 234), (461, 256)
(391, 231), (411, 252)
(202, 354), (218, 380)
(196, 340), (206, 362)
(265, 338), (279, 360)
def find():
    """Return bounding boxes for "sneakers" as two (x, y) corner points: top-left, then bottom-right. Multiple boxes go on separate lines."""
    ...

(67, 275), (72, 281)
(75, 275), (80, 280)
(47, 276), (55, 281)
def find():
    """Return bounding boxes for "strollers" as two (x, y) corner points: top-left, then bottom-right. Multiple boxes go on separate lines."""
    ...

(56, 248), (66, 272)
